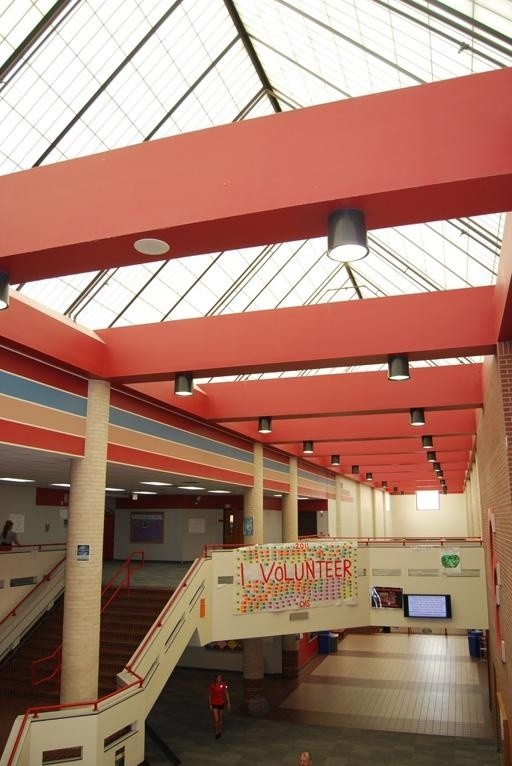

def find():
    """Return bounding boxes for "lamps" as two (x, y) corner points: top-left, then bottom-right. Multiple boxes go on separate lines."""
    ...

(327, 206), (369, 262)
(258, 416), (272, 434)
(410, 409), (425, 426)
(174, 376), (194, 397)
(303, 441), (372, 481)
(387, 355), (411, 382)
(421, 436), (446, 487)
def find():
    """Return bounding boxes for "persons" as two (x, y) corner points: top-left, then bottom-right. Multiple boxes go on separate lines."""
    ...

(0, 520), (21, 551)
(209, 674), (230, 743)
(299, 751), (312, 766)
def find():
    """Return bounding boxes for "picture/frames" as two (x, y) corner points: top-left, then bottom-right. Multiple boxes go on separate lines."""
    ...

(130, 511), (165, 544)
(496, 691), (510, 766)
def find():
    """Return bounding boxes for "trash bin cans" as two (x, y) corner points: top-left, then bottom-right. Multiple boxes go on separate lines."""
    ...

(331, 634), (339, 652)
(318, 631), (331, 655)
(468, 630), (484, 657)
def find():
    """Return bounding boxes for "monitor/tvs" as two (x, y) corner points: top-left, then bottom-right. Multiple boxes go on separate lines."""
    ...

(404, 594), (451, 618)
